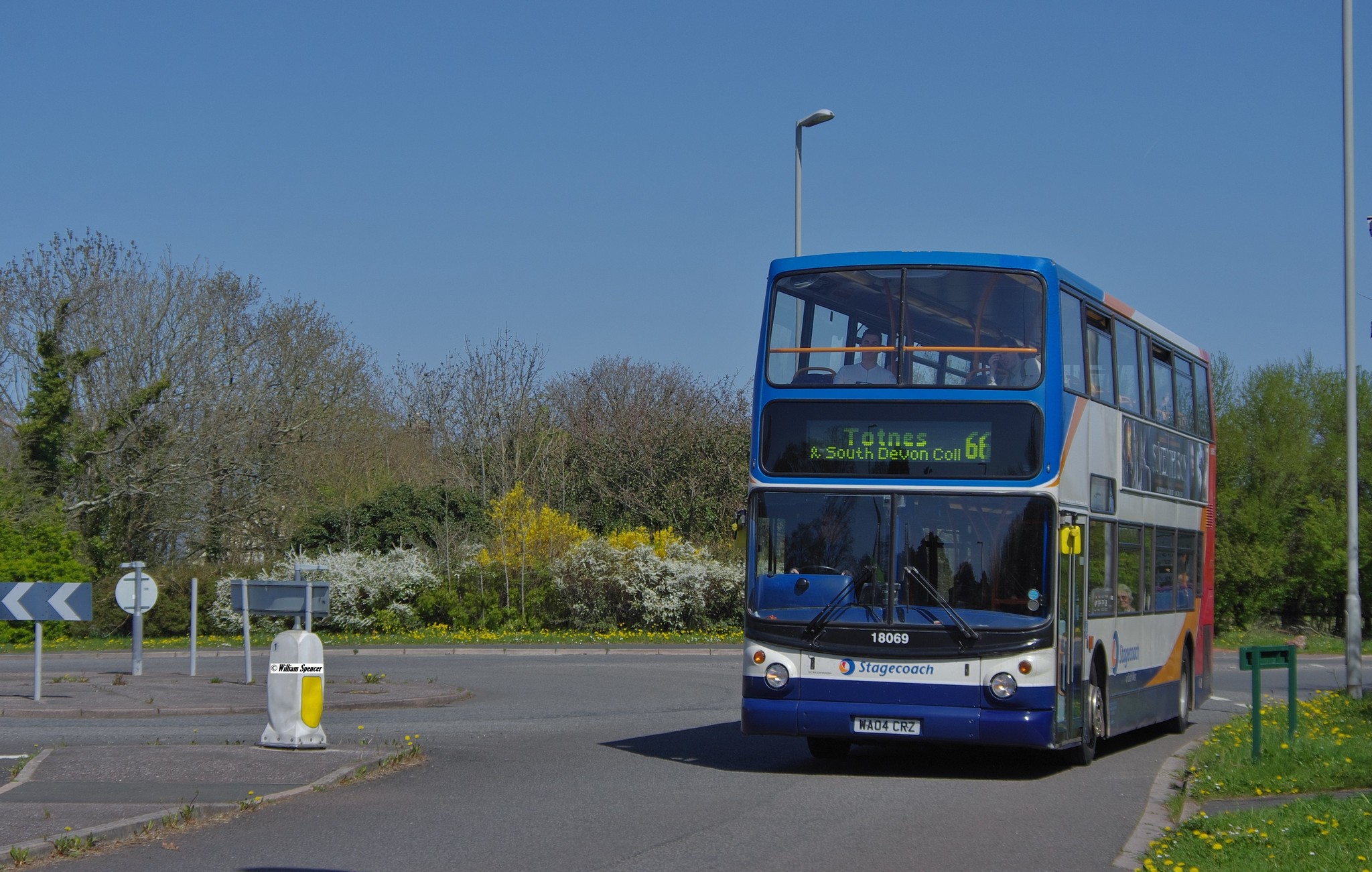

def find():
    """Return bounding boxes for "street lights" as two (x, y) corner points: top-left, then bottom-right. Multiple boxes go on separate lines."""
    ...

(793, 107), (834, 371)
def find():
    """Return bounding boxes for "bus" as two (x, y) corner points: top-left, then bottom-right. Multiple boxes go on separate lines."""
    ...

(731, 250), (1215, 764)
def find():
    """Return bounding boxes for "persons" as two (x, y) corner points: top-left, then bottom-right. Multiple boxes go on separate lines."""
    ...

(789, 511), (855, 576)
(1177, 544), (1192, 586)
(833, 328), (907, 384)
(986, 337), (1041, 385)
(1156, 385), (1173, 421)
(1116, 584), (1135, 612)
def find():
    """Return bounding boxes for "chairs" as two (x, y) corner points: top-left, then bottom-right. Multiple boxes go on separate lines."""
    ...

(964, 368), (1010, 386)
(1150, 583), (1197, 611)
(789, 367), (837, 384)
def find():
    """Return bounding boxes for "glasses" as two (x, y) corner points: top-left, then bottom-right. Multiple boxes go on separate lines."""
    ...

(820, 520), (841, 525)
